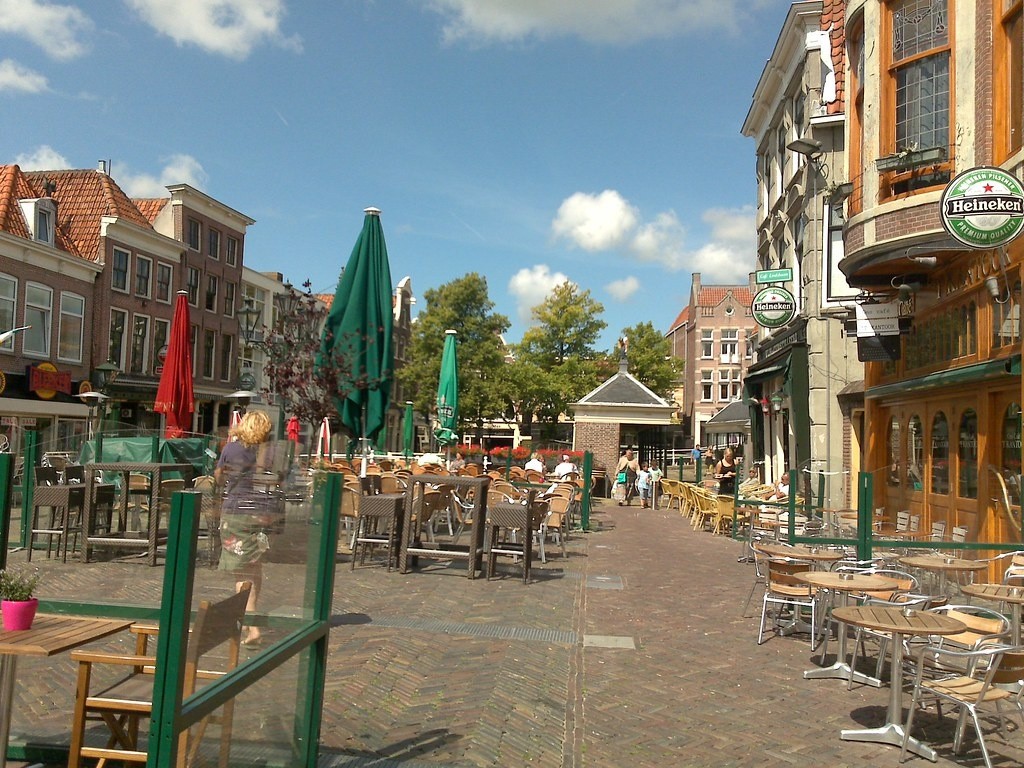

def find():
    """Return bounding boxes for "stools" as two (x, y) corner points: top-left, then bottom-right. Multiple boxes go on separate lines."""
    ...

(63, 463), (116, 559)
(28, 467), (84, 565)
(490, 489), (538, 583)
(351, 477), (406, 573)
(367, 473), (406, 564)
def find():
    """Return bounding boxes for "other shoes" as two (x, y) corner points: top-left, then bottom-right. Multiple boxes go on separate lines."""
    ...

(240, 635), (258, 645)
(641, 504), (645, 508)
(619, 502), (622, 506)
(645, 503), (648, 508)
(627, 502), (631, 506)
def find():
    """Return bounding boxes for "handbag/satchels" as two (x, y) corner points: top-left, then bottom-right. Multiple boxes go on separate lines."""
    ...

(617, 473), (626, 483)
(611, 479), (626, 501)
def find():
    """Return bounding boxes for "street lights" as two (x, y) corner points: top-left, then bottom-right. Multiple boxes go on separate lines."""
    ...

(72, 392), (111, 441)
(93, 358), (122, 464)
(223, 390), (258, 420)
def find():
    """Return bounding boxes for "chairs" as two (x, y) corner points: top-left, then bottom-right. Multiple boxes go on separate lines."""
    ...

(67, 581), (253, 768)
(660, 478), (1024, 768)
(31, 454), (599, 585)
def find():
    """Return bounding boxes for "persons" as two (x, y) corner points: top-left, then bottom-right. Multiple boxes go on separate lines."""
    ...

(524, 451), (578, 481)
(614, 448), (664, 509)
(450, 450), (466, 470)
(758, 471), (790, 510)
(214, 409), (273, 650)
(691, 444), (714, 477)
(716, 448), (737, 495)
(738, 467), (759, 490)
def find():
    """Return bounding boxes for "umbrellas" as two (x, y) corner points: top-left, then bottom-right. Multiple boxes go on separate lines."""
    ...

(315, 207), (394, 479)
(153, 289), (196, 440)
(317, 415), (332, 461)
(433, 330), (459, 471)
(287, 417), (300, 441)
(401, 400), (414, 470)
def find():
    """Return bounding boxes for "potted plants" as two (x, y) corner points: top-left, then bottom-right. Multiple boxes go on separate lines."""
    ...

(0, 568), (40, 630)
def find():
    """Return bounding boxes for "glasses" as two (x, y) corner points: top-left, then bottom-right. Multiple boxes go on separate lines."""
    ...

(749, 470), (755, 473)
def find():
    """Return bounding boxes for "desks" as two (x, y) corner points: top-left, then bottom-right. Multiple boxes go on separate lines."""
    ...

(897, 555), (988, 596)
(82, 462), (193, 568)
(754, 544), (843, 571)
(0, 609), (136, 768)
(399, 474), (490, 580)
(793, 571), (899, 686)
(960, 585), (1024, 653)
(829, 605), (966, 765)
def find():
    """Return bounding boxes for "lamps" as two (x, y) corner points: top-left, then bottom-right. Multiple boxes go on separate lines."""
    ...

(891, 273), (923, 293)
(760, 399), (778, 421)
(983, 273), (1011, 304)
(906, 247), (975, 270)
(771, 395), (789, 419)
(785, 137), (824, 164)
(748, 394), (760, 406)
